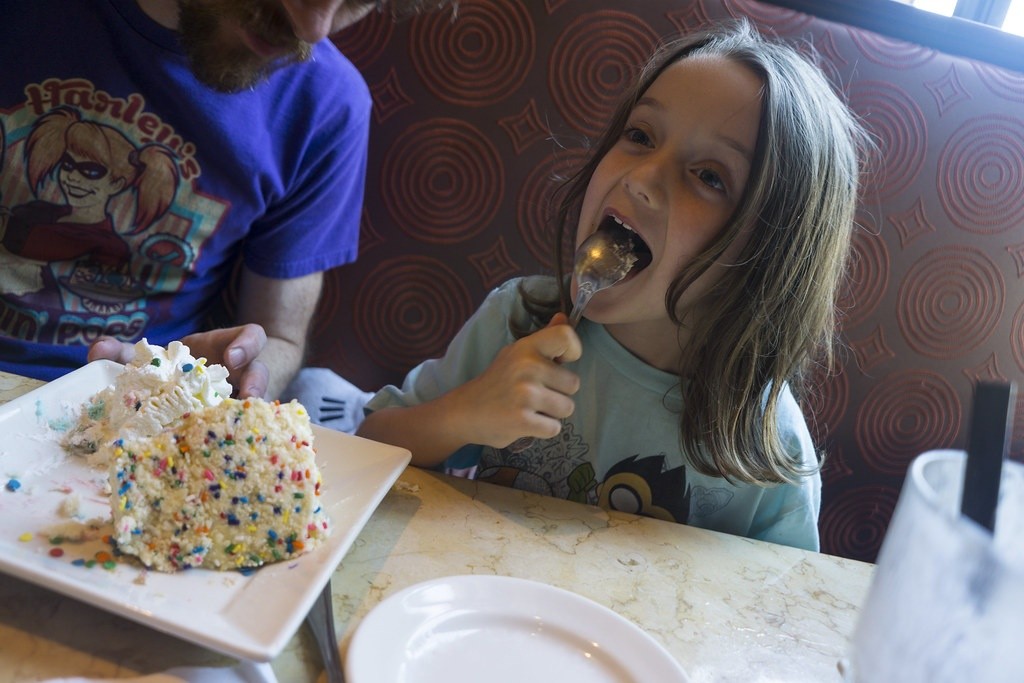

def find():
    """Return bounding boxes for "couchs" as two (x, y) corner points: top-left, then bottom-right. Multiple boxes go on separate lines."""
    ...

(243, 0), (1024, 563)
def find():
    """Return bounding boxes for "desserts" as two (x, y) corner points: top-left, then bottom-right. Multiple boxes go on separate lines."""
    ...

(58, 337), (328, 569)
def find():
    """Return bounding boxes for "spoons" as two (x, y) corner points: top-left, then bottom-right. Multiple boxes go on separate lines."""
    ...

(505, 225), (636, 453)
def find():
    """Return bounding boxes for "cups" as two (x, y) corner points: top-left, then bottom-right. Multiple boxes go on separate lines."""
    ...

(839, 447), (1024, 683)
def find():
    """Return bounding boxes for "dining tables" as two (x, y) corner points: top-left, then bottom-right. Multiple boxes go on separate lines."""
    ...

(0, 371), (877, 683)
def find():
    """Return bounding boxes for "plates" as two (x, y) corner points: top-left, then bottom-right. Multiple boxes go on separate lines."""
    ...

(0, 358), (414, 661)
(317, 574), (690, 683)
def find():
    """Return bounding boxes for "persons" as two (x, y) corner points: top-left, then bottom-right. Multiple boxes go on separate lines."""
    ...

(355, 14), (889, 556)
(0, 1), (378, 400)
